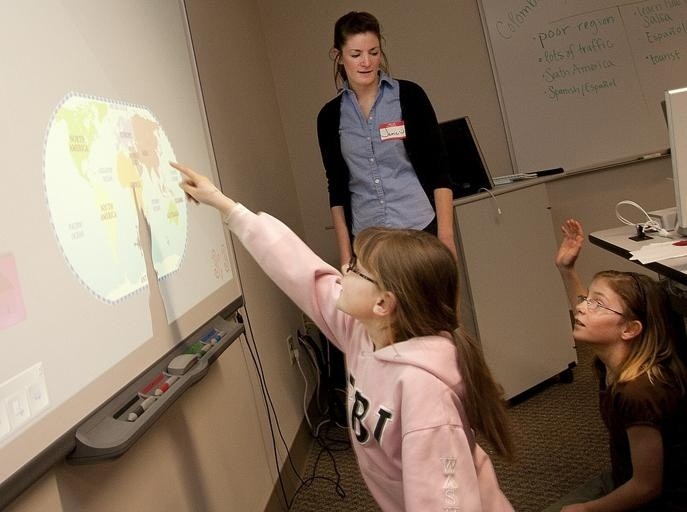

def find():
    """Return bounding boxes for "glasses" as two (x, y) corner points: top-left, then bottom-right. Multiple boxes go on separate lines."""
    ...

(576, 295), (624, 317)
(345, 256), (377, 285)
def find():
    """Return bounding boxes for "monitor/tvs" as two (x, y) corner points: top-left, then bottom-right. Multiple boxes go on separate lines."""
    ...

(439, 114), (495, 200)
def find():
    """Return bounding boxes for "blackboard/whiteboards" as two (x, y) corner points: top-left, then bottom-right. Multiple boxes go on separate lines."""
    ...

(476, 0), (687, 181)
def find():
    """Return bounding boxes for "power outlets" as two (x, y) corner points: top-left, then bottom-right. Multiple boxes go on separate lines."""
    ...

(286, 336), (296, 366)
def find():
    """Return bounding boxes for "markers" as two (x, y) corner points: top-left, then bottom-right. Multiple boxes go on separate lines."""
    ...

(637, 153), (661, 161)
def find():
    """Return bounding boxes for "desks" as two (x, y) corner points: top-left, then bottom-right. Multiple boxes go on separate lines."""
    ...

(589, 222), (687, 287)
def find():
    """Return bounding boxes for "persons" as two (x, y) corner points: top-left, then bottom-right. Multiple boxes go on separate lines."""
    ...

(310, 12), (459, 285)
(543, 217), (687, 512)
(169, 160), (517, 511)
